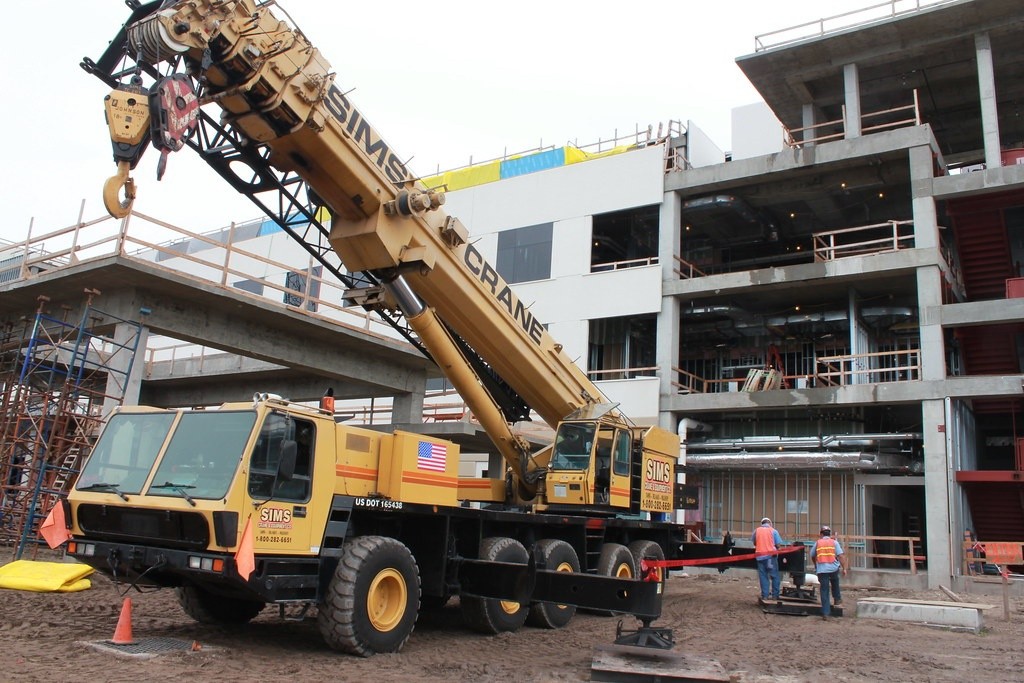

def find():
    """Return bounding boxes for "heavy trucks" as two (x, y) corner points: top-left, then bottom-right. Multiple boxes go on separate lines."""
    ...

(62, 396), (692, 661)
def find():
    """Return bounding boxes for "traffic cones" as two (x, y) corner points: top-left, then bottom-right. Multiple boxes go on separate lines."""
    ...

(104, 596), (139, 646)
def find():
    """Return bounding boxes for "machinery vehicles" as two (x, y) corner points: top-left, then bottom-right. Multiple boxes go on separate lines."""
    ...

(83, 0), (686, 516)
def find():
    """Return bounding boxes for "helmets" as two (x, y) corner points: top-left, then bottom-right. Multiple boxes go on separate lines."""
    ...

(820, 526), (831, 533)
(761, 518), (772, 527)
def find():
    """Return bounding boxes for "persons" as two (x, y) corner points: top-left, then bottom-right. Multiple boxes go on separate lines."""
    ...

(751, 518), (783, 601)
(809, 526), (847, 621)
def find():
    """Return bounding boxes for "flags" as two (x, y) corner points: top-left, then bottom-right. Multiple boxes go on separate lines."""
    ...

(39, 499), (73, 549)
(233, 518), (255, 582)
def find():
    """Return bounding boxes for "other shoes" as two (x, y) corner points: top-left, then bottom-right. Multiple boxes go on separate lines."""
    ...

(773, 596), (779, 601)
(823, 615), (830, 621)
(763, 596), (768, 600)
(834, 600), (842, 605)
